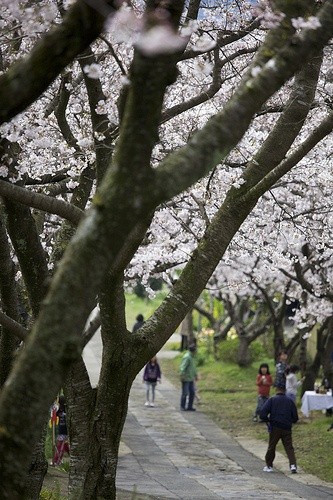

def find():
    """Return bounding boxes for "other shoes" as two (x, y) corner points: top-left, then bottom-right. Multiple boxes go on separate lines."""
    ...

(263, 465), (273, 472)
(150, 402), (155, 406)
(188, 407), (195, 411)
(145, 401), (149, 406)
(290, 465), (296, 472)
(253, 416), (257, 421)
(50, 462), (60, 466)
(260, 419), (263, 422)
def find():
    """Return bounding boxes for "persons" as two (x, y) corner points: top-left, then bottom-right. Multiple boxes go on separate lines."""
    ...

(48, 396), (71, 467)
(143, 354), (162, 406)
(134, 314), (144, 331)
(252, 352), (333, 474)
(180, 316), (200, 411)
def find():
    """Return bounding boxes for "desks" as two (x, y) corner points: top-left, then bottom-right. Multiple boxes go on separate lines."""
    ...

(301, 391), (333, 423)
